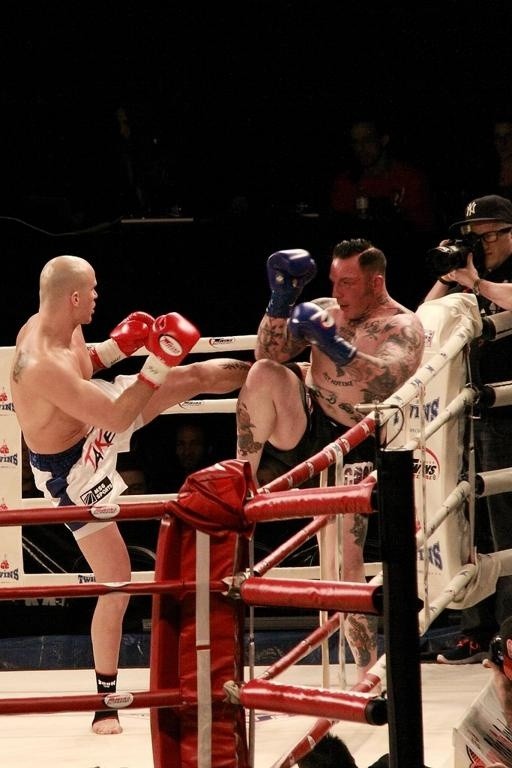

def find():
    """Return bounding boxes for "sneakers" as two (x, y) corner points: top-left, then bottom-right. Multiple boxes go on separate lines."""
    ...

(436, 638), (491, 665)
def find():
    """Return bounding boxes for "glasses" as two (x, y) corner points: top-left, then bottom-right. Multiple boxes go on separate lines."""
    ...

(474, 227), (512, 243)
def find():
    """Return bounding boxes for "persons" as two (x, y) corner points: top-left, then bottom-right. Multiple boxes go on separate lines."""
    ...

(420, 192), (511, 669)
(492, 111), (512, 193)
(235, 236), (420, 696)
(10, 254), (309, 736)
(1, 419), (511, 728)
(115, 106), (135, 143)
(329, 116), (432, 230)
(295, 734), (356, 768)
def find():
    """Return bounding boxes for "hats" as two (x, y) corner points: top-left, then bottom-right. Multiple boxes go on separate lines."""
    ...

(498, 617), (511, 638)
(450, 194), (512, 229)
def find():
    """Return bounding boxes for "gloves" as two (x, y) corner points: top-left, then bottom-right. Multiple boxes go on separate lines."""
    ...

(90, 309), (155, 369)
(267, 248), (316, 318)
(140, 310), (199, 389)
(286, 302), (355, 367)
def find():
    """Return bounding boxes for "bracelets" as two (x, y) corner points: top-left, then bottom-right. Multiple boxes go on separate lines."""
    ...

(473, 279), (486, 298)
(435, 272), (456, 286)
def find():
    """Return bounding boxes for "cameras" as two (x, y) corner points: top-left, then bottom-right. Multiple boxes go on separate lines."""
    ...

(427, 232), (484, 275)
